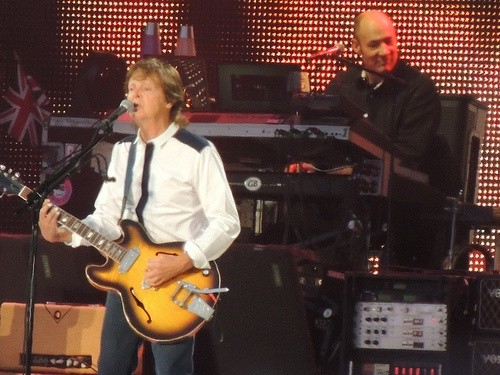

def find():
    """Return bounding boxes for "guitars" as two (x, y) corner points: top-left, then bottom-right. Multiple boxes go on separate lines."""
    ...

(0, 164), (229, 345)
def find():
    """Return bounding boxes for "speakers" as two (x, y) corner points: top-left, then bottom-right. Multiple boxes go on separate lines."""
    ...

(142, 241), (342, 375)
(0, 234), (107, 306)
(432, 93), (489, 273)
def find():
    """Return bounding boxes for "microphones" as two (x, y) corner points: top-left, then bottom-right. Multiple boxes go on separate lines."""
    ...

(101, 99), (134, 126)
(309, 43), (345, 61)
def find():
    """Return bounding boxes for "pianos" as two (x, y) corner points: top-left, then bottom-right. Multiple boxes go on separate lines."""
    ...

(47, 112), (406, 202)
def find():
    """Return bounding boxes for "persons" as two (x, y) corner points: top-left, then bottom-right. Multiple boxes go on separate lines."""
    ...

(282, 9), (441, 250)
(38, 56), (242, 375)
